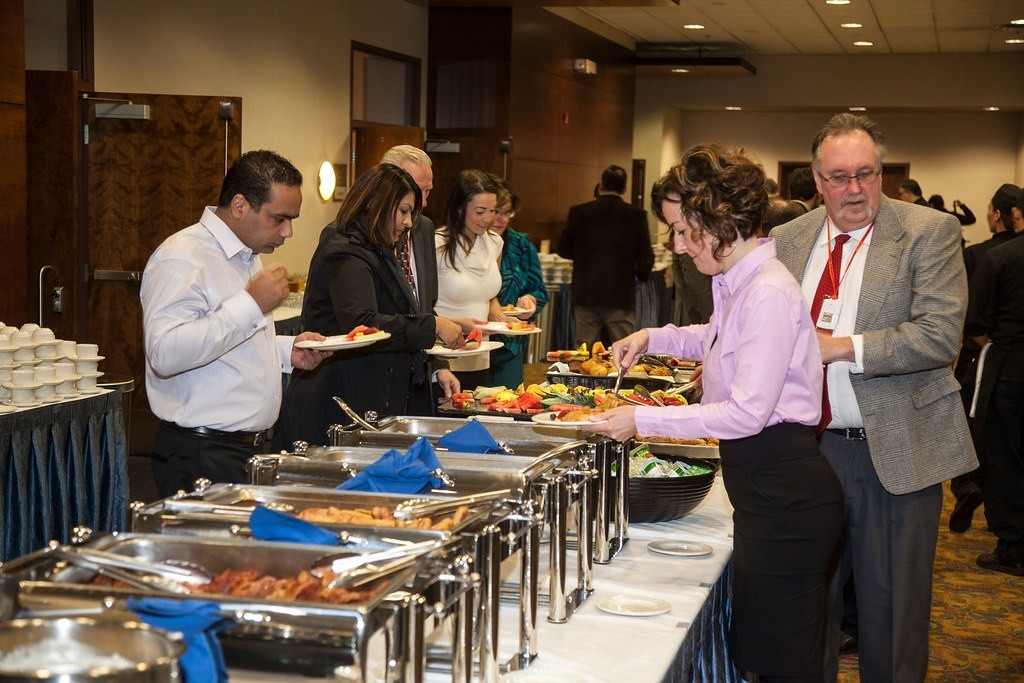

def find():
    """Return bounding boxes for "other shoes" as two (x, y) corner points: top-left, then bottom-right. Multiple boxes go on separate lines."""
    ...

(948, 492), (981, 532)
(976, 553), (1024, 576)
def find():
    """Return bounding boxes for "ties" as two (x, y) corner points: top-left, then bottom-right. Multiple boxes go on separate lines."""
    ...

(396, 233), (414, 289)
(809, 234), (852, 436)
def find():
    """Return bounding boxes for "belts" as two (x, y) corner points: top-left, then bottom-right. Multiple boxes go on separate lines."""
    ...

(827, 428), (866, 440)
(162, 420), (275, 446)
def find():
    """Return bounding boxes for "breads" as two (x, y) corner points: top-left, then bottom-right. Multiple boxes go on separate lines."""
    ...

(581, 360), (673, 377)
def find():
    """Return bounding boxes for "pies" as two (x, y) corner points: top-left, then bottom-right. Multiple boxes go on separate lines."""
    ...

(296, 504), (472, 531)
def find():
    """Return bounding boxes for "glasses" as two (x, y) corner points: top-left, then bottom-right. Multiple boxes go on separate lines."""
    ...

(495, 208), (518, 218)
(816, 168), (882, 187)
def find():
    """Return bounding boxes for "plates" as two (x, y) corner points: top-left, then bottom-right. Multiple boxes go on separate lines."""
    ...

(474, 320), (542, 335)
(67, 356), (106, 360)
(41, 339), (62, 345)
(647, 540), (713, 555)
(80, 372), (105, 378)
(32, 356), (64, 362)
(77, 387), (104, 393)
(3, 382), (42, 390)
(500, 307), (530, 315)
(14, 360), (41, 365)
(530, 410), (609, 425)
(6, 402), (41, 407)
(294, 333), (391, 351)
(596, 594), (673, 616)
(425, 341), (504, 357)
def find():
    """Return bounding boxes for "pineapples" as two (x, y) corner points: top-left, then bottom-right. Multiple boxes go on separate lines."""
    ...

(493, 383), (605, 401)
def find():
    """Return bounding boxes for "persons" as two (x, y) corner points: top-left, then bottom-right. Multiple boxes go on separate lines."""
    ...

(949, 183), (1024, 577)
(140, 149), (334, 498)
(767, 113), (981, 683)
(581, 142), (843, 683)
(278, 144), (549, 451)
(554, 164), (977, 350)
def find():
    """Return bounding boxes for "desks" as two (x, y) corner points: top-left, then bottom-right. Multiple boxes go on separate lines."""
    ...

(0, 373), (135, 565)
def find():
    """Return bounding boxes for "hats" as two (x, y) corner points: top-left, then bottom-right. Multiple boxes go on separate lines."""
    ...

(992, 183), (1024, 214)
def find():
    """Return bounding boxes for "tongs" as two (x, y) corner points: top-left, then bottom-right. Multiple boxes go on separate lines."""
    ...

(464, 340), (480, 350)
(164, 498), (296, 516)
(49, 541), (213, 594)
(666, 380), (696, 396)
(605, 384), (665, 407)
(395, 489), (511, 521)
(309, 540), (441, 589)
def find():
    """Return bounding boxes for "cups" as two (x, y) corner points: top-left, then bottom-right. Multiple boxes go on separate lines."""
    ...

(536, 251), (572, 284)
(0, 320), (98, 404)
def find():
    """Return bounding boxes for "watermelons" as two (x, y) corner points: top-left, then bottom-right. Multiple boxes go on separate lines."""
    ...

(626, 393), (682, 406)
(466, 327), (482, 347)
(345, 325), (372, 342)
(451, 391), (546, 414)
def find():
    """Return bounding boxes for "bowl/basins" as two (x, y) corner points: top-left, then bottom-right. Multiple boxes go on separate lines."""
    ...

(634, 437), (722, 467)
(579, 453), (719, 522)
(650, 391), (688, 408)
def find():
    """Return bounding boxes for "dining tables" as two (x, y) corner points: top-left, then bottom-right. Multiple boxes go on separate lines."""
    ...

(421, 466), (747, 683)
